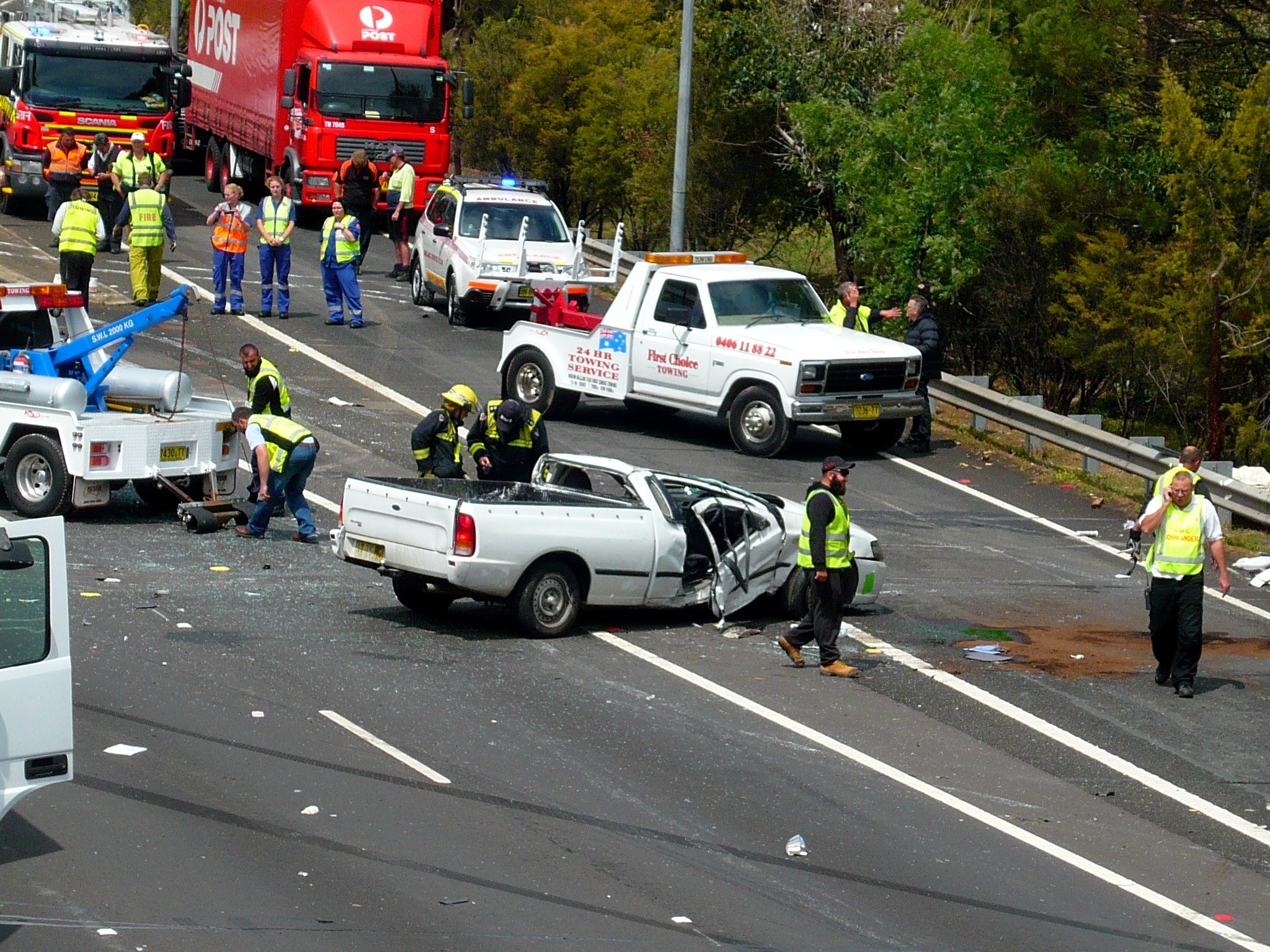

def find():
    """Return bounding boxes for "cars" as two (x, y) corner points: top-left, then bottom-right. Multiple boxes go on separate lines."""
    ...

(409, 175), (592, 327)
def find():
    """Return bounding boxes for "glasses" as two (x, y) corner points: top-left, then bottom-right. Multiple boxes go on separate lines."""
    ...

(830, 468), (850, 477)
(385, 155), (394, 161)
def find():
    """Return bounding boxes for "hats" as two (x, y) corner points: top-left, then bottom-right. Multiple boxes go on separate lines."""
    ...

(497, 399), (522, 432)
(130, 132), (145, 141)
(383, 145), (405, 158)
(821, 456), (856, 472)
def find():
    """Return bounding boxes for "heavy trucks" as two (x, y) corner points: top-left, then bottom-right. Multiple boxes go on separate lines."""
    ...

(1, 1), (194, 215)
(183, 0), (476, 225)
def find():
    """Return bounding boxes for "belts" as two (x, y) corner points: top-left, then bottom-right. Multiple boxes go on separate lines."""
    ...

(298, 443), (317, 447)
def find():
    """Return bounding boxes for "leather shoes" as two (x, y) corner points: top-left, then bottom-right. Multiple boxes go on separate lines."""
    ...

(293, 533), (319, 544)
(235, 525), (264, 539)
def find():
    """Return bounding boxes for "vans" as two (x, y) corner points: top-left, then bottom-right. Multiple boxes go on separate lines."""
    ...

(0, 516), (75, 822)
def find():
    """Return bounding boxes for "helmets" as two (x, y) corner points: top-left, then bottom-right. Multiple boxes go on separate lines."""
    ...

(441, 384), (478, 415)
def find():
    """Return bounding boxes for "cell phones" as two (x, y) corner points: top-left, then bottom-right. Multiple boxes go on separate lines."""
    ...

(225, 211), (234, 214)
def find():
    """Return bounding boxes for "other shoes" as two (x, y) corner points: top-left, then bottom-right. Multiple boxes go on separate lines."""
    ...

(325, 319), (344, 325)
(270, 505), (285, 517)
(96, 241), (121, 253)
(354, 265), (361, 275)
(350, 322), (362, 328)
(135, 298), (156, 306)
(48, 237), (59, 247)
(211, 308), (289, 319)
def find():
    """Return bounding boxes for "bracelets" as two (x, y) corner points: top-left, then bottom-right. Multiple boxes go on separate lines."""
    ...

(279, 237), (284, 242)
(342, 227), (346, 230)
(158, 183), (162, 187)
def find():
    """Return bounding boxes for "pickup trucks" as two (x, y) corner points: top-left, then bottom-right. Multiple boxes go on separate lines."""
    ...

(329, 453), (888, 638)
(1, 283), (243, 518)
(495, 249), (924, 457)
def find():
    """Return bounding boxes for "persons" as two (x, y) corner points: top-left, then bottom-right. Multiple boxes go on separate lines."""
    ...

(410, 384), (482, 479)
(1141, 472), (1230, 697)
(319, 198), (363, 328)
(333, 151), (382, 274)
(256, 177), (296, 319)
(897, 296), (942, 451)
(232, 407), (319, 544)
(1126, 446), (1219, 570)
(52, 188), (104, 312)
(111, 132), (168, 260)
(206, 184), (252, 315)
(88, 133), (126, 253)
(466, 400), (550, 483)
(112, 172), (177, 306)
(383, 146), (415, 280)
(42, 127), (89, 247)
(778, 455), (860, 676)
(830, 281), (902, 332)
(240, 344), (292, 516)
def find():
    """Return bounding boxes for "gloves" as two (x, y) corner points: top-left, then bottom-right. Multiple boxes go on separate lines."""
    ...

(1126, 528), (1142, 561)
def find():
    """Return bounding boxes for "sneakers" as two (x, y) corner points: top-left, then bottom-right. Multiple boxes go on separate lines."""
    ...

(397, 271), (410, 281)
(1155, 663), (1171, 684)
(819, 660), (859, 677)
(777, 636), (806, 667)
(385, 269), (403, 277)
(1179, 684), (1194, 697)
